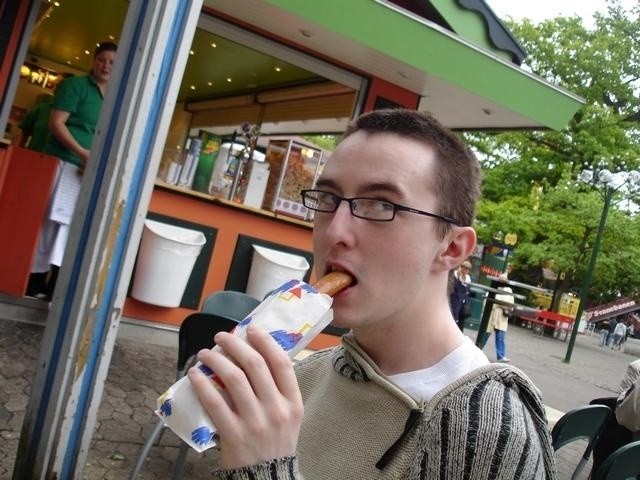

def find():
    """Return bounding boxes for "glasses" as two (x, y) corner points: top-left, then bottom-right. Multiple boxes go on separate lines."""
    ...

(299, 188), (461, 227)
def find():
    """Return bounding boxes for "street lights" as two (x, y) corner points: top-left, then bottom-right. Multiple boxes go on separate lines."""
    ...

(560, 168), (640, 365)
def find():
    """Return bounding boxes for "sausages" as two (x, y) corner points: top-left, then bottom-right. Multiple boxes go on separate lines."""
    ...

(312, 271), (354, 297)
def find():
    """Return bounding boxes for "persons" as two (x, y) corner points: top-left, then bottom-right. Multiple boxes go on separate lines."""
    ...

(604, 315), (617, 346)
(182, 106), (563, 480)
(27, 39), (119, 303)
(594, 357), (639, 471)
(15, 72), (76, 149)
(611, 318), (626, 352)
(482, 271), (512, 364)
(617, 322), (634, 353)
(452, 258), (475, 336)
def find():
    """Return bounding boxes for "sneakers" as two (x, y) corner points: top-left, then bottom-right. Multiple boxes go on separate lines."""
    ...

(496, 357), (510, 362)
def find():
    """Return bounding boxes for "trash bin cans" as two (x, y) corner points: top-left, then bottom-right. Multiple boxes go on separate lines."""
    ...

(463, 288), (489, 330)
(246, 243), (310, 303)
(130, 219), (207, 309)
